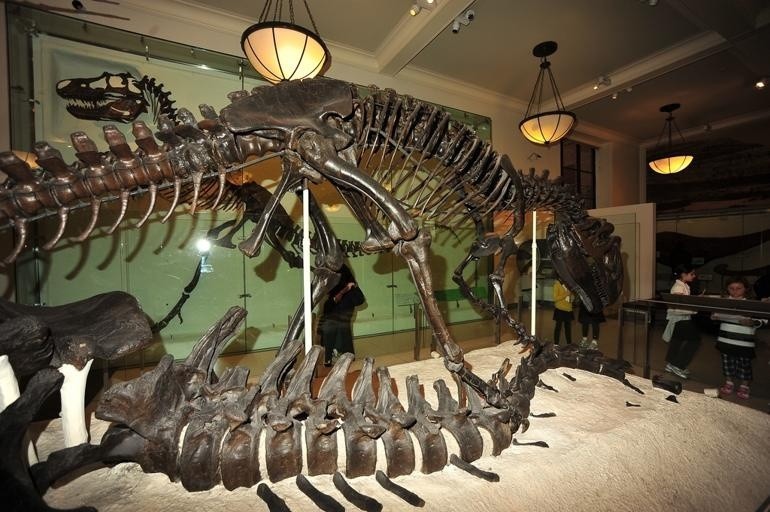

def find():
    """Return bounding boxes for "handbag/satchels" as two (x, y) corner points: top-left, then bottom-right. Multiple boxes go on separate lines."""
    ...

(342, 286), (365, 306)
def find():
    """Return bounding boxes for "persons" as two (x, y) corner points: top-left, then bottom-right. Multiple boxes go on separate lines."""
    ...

(553, 279), (575, 345)
(711, 275), (768, 399)
(578, 303), (606, 349)
(317, 264), (365, 367)
(664, 263), (701, 379)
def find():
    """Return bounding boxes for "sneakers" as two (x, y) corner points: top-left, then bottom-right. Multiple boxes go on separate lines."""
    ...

(578, 338), (588, 348)
(719, 381), (735, 395)
(736, 384), (750, 399)
(588, 341), (600, 349)
(665, 364), (690, 379)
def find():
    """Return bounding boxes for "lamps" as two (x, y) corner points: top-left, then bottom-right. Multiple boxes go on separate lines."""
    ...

(519, 42), (577, 144)
(410, 0), (474, 33)
(241, 0), (328, 84)
(648, 103), (694, 175)
